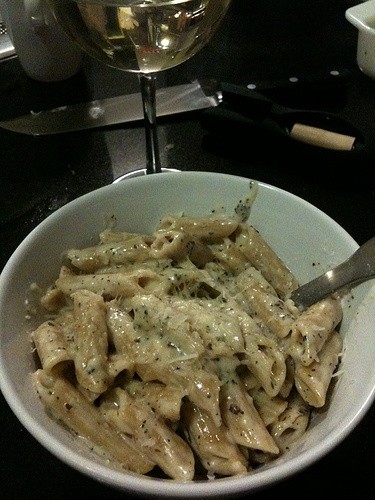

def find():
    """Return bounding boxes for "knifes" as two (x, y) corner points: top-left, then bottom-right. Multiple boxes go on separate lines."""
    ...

(0, 65), (353, 136)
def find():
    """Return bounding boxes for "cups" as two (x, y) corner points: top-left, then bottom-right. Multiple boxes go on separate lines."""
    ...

(0, 0), (85, 82)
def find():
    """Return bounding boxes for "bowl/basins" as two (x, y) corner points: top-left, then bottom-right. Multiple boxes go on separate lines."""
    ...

(0, 171), (375, 499)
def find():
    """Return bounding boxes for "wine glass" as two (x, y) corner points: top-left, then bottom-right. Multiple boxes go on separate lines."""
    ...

(48, 0), (231, 184)
